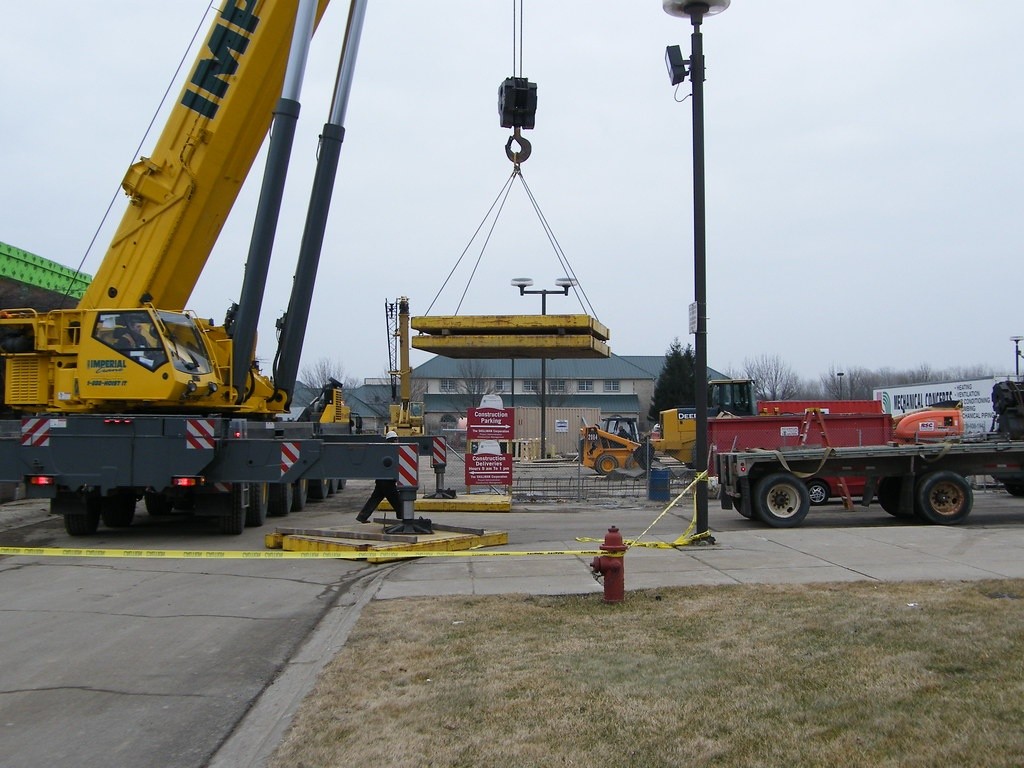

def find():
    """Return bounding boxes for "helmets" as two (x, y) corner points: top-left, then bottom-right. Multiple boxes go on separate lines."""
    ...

(385, 430), (398, 440)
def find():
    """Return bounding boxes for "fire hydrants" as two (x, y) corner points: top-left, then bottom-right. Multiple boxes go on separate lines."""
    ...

(590, 526), (629, 606)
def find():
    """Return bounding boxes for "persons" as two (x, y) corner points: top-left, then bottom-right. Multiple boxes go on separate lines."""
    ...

(356, 431), (401, 523)
(114, 315), (148, 349)
(617, 426), (627, 438)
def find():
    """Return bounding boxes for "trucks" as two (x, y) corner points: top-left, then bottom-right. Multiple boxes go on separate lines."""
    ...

(715, 381), (1024, 530)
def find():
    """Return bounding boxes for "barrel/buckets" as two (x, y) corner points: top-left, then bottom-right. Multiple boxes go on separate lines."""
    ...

(650, 468), (670, 501)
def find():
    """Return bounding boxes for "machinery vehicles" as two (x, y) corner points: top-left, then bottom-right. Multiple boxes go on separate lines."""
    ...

(578, 378), (967, 503)
(1, 0), (613, 539)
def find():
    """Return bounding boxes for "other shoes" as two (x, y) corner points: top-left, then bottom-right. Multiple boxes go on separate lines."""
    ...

(355, 517), (371, 523)
